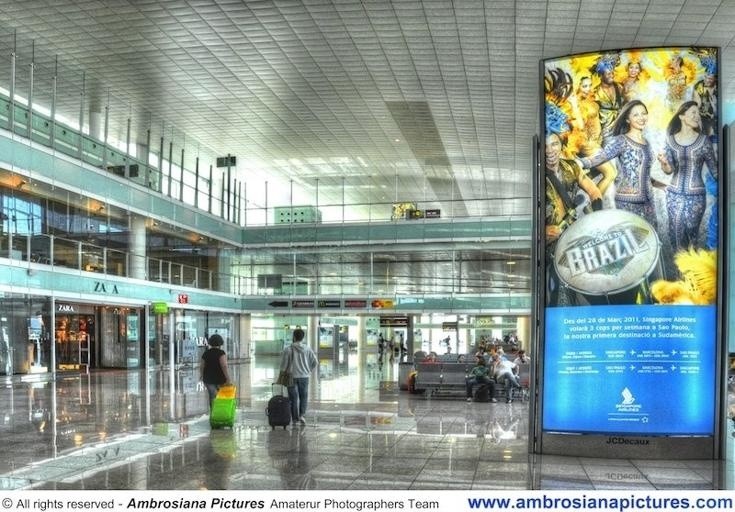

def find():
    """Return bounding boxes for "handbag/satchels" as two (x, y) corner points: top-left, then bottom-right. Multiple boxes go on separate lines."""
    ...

(281, 372), (293, 387)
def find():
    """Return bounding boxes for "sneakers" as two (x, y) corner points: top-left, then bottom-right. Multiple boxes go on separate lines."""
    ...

(300, 417), (305, 423)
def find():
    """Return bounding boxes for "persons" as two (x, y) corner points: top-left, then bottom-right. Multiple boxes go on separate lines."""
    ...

(466, 355), (498, 403)
(376, 332), (385, 362)
(457, 334), (531, 365)
(388, 336), (395, 355)
(545, 133), (601, 306)
(399, 333), (407, 353)
(487, 353), (522, 403)
(576, 76), (618, 214)
(279, 329), (319, 426)
(199, 334), (234, 408)
(576, 99), (664, 280)
(691, 73), (717, 136)
(593, 65), (623, 139)
(620, 60), (642, 99)
(658, 101), (718, 253)
(663, 56), (689, 113)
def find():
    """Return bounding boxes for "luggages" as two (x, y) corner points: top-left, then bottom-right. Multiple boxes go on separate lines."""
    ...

(210, 384), (237, 429)
(268, 383), (290, 429)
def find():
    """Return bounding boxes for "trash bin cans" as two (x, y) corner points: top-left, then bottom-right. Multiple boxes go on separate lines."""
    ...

(399, 362), (415, 390)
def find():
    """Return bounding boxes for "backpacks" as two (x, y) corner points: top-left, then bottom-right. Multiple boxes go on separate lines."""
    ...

(475, 385), (488, 402)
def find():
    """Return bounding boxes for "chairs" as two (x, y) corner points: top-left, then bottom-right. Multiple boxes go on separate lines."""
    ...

(414, 361), (530, 401)
